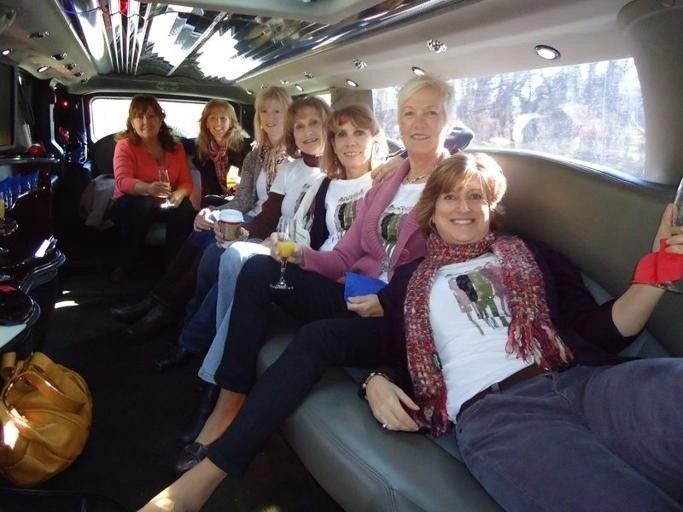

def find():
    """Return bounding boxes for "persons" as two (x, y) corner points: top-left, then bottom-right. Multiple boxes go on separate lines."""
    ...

(154, 97), (332, 372)
(192, 101), (251, 216)
(358, 152), (683, 510)
(179, 106), (386, 445)
(135, 74), (455, 511)
(108, 95), (196, 296)
(110, 85), (293, 370)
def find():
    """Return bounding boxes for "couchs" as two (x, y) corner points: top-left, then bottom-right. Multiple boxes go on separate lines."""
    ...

(88, 130), (201, 266)
(256, 139), (683, 511)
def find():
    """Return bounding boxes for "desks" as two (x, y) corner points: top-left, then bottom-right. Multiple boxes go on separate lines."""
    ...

(0, 229), (65, 380)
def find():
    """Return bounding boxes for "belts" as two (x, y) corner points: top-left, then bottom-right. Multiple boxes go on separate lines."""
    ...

(456, 360), (546, 421)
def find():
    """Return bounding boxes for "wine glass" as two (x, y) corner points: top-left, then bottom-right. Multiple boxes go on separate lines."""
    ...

(269, 216), (296, 290)
(655, 175), (682, 293)
(220, 166), (239, 199)
(158, 168), (175, 207)
(0, 192), (5, 219)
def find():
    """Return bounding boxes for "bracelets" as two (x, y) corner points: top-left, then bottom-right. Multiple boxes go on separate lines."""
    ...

(363, 373), (391, 402)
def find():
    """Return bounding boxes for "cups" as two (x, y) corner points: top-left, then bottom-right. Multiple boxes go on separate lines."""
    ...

(219, 209), (243, 248)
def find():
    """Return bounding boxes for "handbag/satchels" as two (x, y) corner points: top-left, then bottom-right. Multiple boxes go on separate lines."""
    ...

(0, 352), (93, 487)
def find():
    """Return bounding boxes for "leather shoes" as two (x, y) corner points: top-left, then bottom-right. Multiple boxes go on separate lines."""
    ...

(156, 344), (187, 372)
(176, 442), (207, 476)
(127, 304), (174, 340)
(110, 301), (152, 322)
(196, 384), (220, 422)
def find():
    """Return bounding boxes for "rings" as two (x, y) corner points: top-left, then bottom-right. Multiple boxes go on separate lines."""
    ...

(380, 422), (387, 429)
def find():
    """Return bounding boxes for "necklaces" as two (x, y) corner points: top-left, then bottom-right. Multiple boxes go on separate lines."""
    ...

(401, 168), (432, 185)
